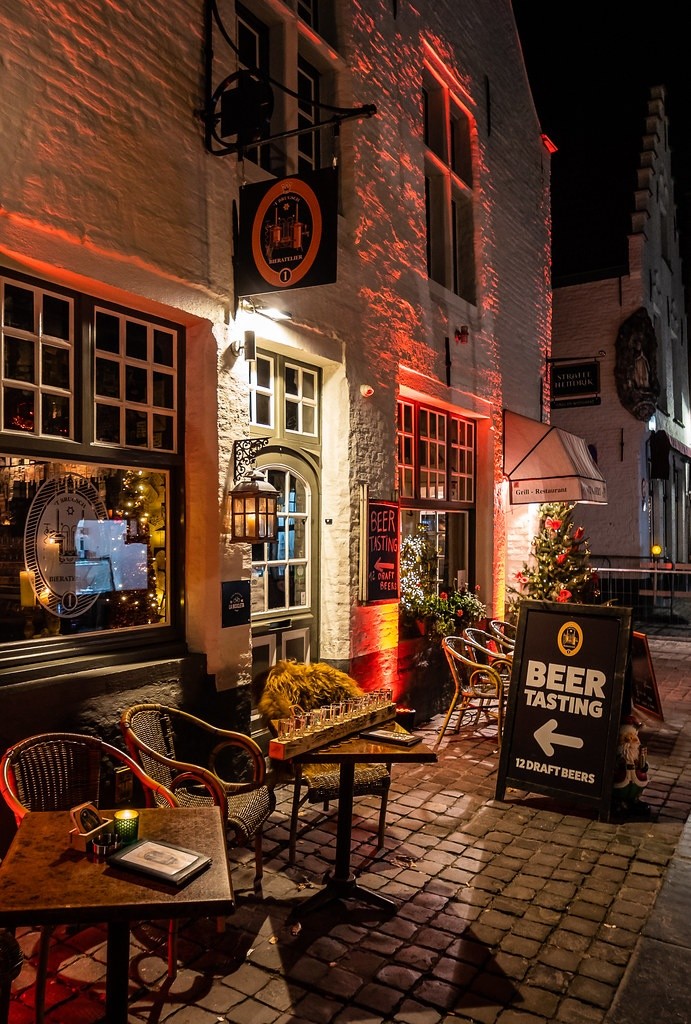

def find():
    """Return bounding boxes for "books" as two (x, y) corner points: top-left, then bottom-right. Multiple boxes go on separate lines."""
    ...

(112, 838), (212, 886)
(359, 728), (424, 747)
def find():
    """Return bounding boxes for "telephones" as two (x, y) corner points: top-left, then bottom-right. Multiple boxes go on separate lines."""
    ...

(626, 630), (663, 720)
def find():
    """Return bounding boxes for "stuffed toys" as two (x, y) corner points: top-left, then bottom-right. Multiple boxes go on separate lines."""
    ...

(615, 715), (649, 822)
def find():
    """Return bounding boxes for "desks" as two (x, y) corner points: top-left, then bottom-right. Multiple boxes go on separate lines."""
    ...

(0, 804), (235, 1023)
(271, 716), (440, 927)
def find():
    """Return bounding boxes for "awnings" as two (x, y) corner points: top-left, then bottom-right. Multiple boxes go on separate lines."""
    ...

(503, 408), (608, 505)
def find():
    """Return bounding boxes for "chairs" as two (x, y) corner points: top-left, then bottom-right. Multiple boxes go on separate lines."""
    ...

(434, 620), (520, 749)
(1, 732), (182, 979)
(121, 702), (276, 912)
(264, 664), (391, 865)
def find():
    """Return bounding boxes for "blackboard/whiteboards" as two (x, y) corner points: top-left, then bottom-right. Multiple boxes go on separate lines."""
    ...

(363, 498), (400, 607)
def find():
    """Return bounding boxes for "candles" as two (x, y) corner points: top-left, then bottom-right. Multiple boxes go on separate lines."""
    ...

(19, 568), (36, 604)
(40, 589), (50, 605)
(44, 539), (59, 569)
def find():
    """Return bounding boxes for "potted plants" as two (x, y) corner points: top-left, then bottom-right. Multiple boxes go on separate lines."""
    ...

(410, 589), (486, 634)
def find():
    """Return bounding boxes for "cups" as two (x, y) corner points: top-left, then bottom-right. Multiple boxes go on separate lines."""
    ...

(278, 688), (392, 740)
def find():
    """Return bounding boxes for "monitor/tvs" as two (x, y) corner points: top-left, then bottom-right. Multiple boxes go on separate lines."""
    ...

(73, 558), (116, 596)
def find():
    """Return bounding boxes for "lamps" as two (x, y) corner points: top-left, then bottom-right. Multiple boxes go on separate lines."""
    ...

(229, 329), (258, 362)
(226, 469), (282, 546)
(454, 324), (469, 344)
